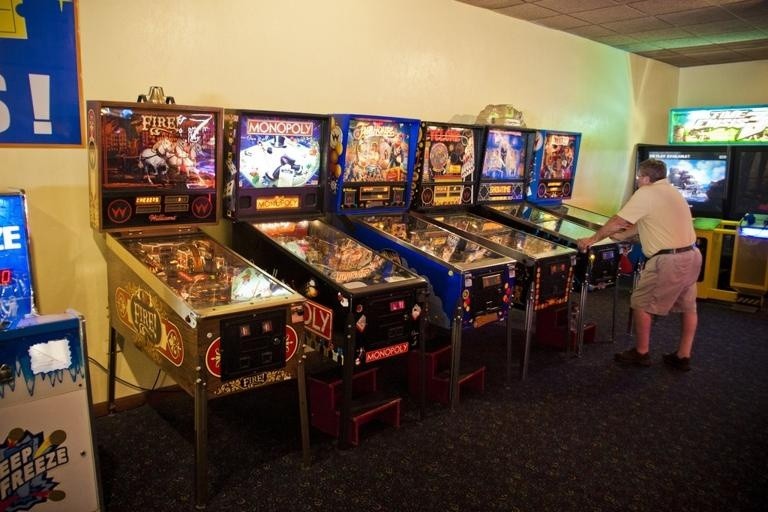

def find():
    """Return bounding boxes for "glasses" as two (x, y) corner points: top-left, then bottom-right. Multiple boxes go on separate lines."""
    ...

(635, 175), (643, 180)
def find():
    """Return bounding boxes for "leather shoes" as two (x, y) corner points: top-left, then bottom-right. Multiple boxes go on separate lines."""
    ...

(614, 348), (651, 368)
(662, 350), (694, 371)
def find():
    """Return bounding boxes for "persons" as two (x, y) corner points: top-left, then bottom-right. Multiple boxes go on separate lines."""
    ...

(577, 159), (704, 373)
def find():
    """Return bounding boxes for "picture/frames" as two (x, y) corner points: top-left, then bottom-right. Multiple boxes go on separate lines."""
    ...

(0, 0), (87, 150)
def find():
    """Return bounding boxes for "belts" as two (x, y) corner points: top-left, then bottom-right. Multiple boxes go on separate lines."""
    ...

(655, 246), (694, 254)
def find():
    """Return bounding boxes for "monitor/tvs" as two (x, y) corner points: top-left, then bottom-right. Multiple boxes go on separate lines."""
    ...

(730, 141), (768, 213)
(634, 142), (729, 219)
